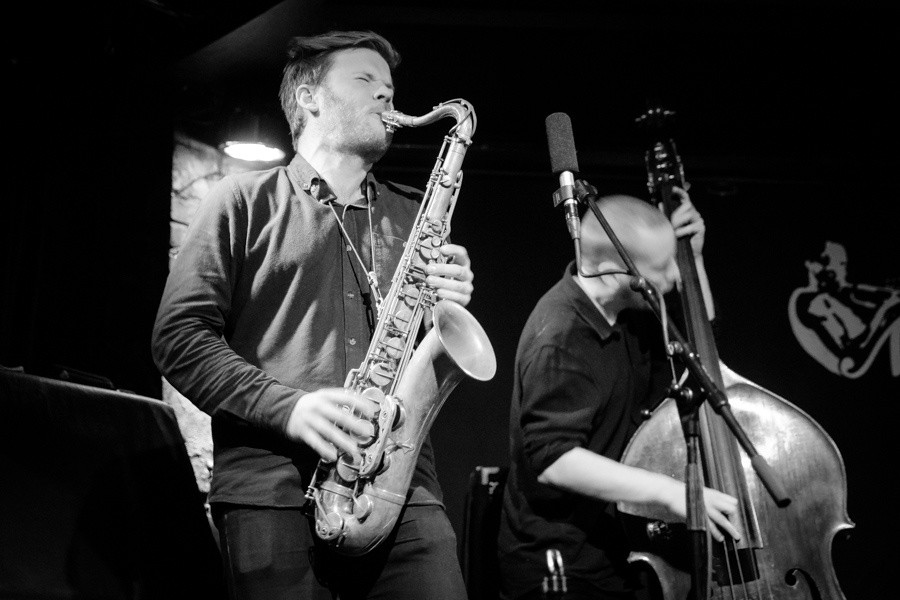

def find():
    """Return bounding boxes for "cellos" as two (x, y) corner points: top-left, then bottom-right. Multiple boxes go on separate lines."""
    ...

(620, 107), (856, 600)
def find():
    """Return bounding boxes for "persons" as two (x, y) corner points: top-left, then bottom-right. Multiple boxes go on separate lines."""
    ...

(151, 32), (475, 600)
(494, 194), (716, 600)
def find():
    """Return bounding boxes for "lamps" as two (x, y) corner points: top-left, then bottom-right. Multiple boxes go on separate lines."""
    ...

(217, 91), (288, 169)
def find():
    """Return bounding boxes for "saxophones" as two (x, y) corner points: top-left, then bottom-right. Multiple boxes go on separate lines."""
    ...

(306, 96), (498, 554)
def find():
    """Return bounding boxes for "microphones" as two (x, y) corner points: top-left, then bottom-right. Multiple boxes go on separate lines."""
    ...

(545, 112), (582, 243)
